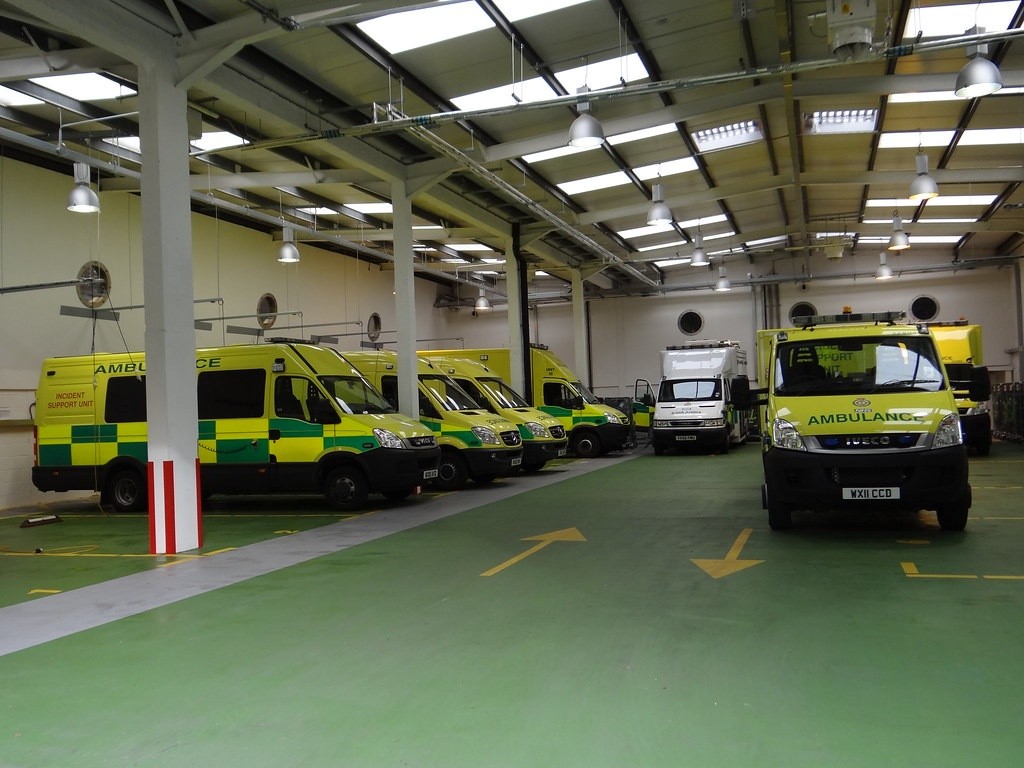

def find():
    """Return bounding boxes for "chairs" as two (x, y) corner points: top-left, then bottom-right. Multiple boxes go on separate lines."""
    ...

(273, 375), (308, 424)
(305, 382), (341, 425)
(783, 344), (826, 387)
(872, 343), (905, 381)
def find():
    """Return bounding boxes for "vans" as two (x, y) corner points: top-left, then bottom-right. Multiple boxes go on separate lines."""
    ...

(425, 356), (568, 476)
(339, 350), (523, 493)
(31, 342), (439, 514)
(755, 323), (972, 546)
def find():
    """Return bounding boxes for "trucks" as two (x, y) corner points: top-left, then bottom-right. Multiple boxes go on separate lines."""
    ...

(930, 323), (992, 459)
(395, 344), (636, 459)
(633, 338), (755, 457)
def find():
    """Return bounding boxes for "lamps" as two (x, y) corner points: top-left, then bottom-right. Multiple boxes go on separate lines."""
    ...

(646, 163), (673, 226)
(66, 160), (100, 213)
(954, 23), (1003, 98)
(566, 55), (604, 150)
(875, 239), (894, 280)
(475, 273), (489, 310)
(715, 255), (733, 292)
(887, 207), (911, 251)
(690, 218), (711, 267)
(272, 226), (300, 263)
(908, 131), (939, 201)
